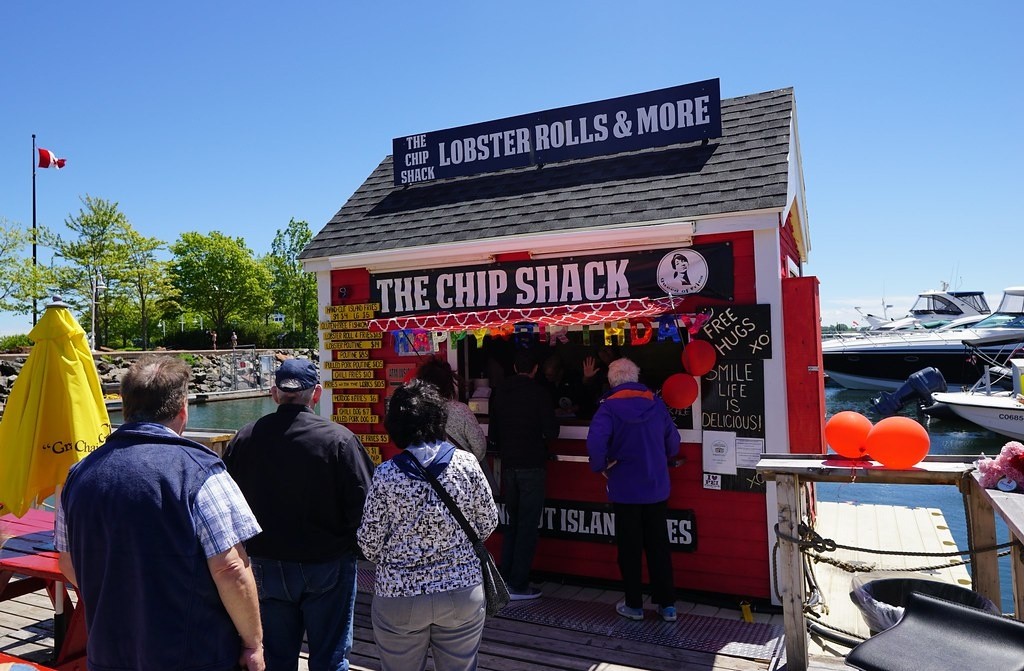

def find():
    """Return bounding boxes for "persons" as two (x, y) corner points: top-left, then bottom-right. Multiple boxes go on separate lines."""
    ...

(211, 331), (217, 350)
(221, 358), (375, 671)
(231, 332), (237, 351)
(357, 379), (499, 671)
(672, 253), (691, 287)
(586, 359), (682, 620)
(61, 356), (266, 671)
(539, 359), (581, 418)
(488, 350), (561, 599)
(418, 359), (487, 465)
(579, 339), (622, 420)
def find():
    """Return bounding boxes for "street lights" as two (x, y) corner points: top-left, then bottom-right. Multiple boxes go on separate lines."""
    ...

(90, 274), (108, 354)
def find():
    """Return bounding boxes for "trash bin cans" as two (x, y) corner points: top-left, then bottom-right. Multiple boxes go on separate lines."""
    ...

(849, 577), (999, 638)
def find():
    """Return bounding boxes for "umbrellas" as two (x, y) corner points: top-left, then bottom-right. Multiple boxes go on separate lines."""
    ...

(0, 294), (113, 661)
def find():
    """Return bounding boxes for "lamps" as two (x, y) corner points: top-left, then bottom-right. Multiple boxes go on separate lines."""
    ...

(367, 254), (495, 274)
(529, 237), (693, 259)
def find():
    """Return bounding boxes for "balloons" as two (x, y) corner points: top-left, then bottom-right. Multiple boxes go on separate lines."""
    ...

(824, 411), (930, 467)
(663, 340), (716, 409)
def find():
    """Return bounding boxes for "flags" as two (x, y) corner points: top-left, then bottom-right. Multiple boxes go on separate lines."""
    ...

(38, 148), (67, 169)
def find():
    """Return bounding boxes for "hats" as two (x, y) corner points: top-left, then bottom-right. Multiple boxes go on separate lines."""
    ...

(275, 358), (320, 392)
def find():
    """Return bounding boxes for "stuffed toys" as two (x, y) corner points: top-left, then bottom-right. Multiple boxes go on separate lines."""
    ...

(977, 441), (1024, 488)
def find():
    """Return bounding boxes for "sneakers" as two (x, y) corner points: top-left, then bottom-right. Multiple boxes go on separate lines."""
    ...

(506, 584), (542, 600)
(656, 604), (677, 620)
(616, 602), (644, 619)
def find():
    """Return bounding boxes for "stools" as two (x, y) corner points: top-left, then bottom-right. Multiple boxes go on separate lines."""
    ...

(844, 592), (1024, 671)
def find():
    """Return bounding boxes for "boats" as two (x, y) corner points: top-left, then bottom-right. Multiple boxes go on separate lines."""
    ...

(869, 358), (1024, 443)
(819, 285), (1023, 396)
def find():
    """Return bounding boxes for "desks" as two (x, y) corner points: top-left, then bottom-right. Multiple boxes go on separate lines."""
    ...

(0, 508), (88, 671)
(756, 451), (1024, 671)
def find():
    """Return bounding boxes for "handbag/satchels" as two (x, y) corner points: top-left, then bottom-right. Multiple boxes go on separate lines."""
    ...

(479, 547), (510, 615)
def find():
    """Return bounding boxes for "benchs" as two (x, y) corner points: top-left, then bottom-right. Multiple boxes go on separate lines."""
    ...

(0, 653), (60, 671)
